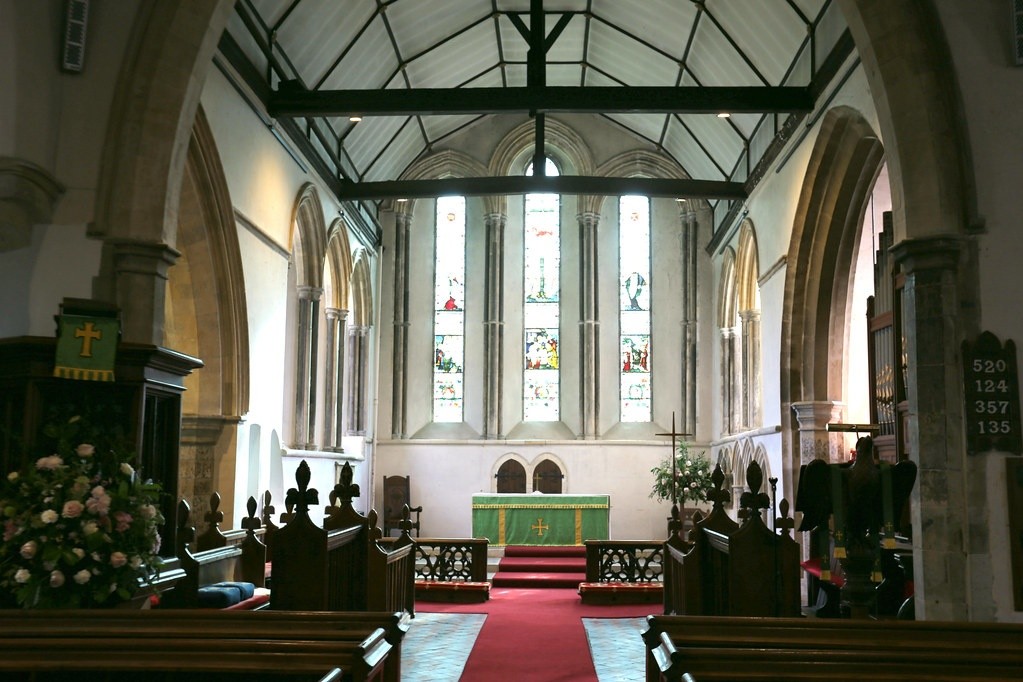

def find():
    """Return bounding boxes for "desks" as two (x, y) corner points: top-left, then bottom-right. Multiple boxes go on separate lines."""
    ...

(473, 492), (611, 548)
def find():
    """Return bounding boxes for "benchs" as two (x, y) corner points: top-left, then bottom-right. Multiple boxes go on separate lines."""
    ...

(0, 492), (408, 682)
(643, 614), (1023, 682)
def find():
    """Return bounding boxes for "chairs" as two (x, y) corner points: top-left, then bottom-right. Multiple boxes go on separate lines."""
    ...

(680, 508), (710, 541)
(384, 474), (422, 536)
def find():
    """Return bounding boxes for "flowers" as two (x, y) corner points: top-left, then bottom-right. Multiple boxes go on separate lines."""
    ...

(0, 421), (171, 609)
(649, 442), (717, 504)
